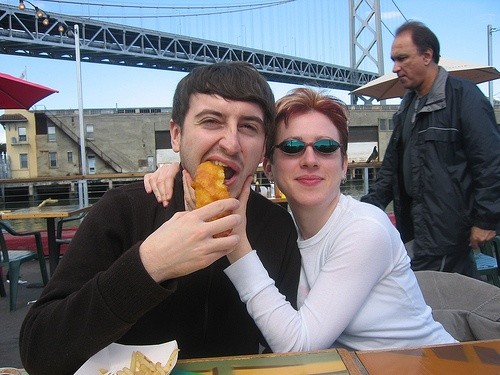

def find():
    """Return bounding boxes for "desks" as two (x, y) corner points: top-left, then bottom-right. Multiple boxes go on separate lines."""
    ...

(0, 203), (91, 279)
(173, 339), (500, 375)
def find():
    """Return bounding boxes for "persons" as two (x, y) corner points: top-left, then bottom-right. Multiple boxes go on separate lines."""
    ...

(19, 61), (300, 375)
(360, 20), (500, 281)
(143, 89), (459, 354)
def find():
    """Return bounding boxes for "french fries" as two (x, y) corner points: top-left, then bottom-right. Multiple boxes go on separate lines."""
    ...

(99, 349), (180, 375)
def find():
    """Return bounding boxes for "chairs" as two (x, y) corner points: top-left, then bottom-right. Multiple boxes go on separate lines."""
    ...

(0, 222), (49, 312)
(413, 270), (500, 342)
(56, 208), (89, 267)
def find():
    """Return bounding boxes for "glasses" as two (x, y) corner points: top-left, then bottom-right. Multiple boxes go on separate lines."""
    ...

(271, 139), (344, 155)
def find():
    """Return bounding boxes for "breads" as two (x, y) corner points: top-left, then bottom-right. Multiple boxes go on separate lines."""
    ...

(192, 161), (234, 237)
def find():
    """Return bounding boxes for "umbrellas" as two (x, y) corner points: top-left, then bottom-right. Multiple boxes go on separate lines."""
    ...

(348, 58), (500, 102)
(0, 73), (59, 110)
